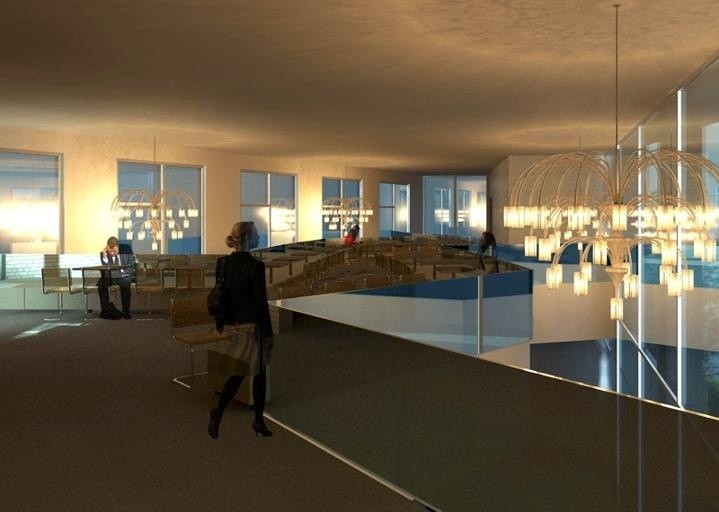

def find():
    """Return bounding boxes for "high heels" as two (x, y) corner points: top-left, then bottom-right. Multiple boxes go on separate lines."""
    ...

(252, 420), (273, 437)
(207, 408), (222, 439)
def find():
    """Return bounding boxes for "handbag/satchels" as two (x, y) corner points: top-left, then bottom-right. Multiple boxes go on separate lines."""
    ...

(206, 280), (228, 319)
(102, 302), (124, 320)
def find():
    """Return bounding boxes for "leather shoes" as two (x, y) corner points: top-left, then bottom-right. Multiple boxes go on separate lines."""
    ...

(125, 313), (131, 319)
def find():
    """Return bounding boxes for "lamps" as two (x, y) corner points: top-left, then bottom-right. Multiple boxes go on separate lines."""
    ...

(110, 136), (199, 251)
(503, 4), (719, 320)
(321, 196), (374, 237)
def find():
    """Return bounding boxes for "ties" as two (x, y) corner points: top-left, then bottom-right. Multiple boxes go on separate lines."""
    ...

(115, 255), (119, 264)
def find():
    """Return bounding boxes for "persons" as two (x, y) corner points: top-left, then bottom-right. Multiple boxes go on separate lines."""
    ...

(472, 231), (499, 276)
(345, 225), (362, 246)
(208, 221), (273, 440)
(98, 237), (137, 319)
